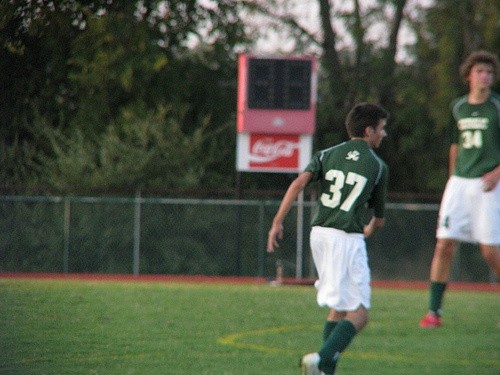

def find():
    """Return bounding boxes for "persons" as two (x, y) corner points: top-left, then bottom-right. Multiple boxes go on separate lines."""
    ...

(417, 49), (500, 329)
(265, 101), (389, 375)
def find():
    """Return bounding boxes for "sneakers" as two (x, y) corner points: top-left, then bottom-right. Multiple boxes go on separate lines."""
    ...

(299, 350), (322, 374)
(419, 312), (442, 328)
